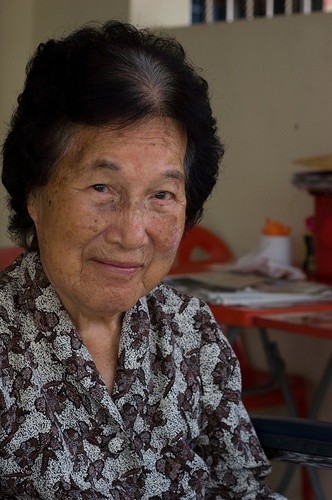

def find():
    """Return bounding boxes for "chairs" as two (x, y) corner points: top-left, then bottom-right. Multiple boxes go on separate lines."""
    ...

(168, 226), (315, 500)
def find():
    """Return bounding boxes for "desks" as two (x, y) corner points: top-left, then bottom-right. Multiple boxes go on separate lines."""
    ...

(210, 301), (332, 500)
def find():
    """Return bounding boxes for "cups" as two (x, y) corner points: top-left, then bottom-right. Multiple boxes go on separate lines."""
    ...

(261, 218), (290, 270)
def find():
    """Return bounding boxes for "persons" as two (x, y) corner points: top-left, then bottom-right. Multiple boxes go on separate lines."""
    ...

(0, 17), (287, 500)
